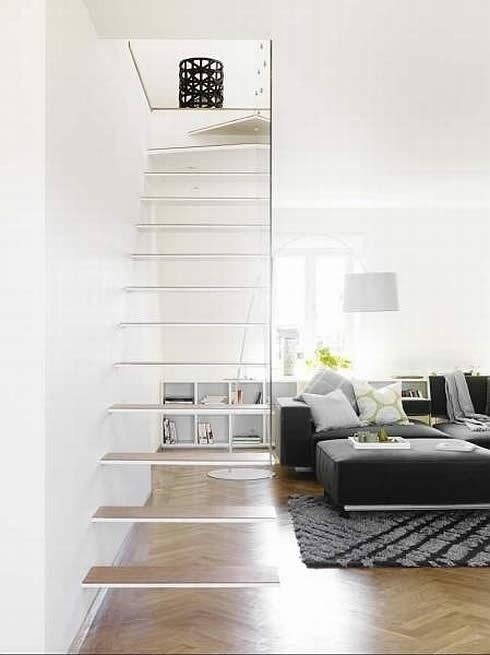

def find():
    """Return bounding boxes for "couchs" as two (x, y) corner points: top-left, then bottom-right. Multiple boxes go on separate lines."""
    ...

(278, 368), (490, 474)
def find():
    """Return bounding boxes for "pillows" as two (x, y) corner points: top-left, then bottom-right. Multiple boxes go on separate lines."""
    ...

(292, 368), (415, 432)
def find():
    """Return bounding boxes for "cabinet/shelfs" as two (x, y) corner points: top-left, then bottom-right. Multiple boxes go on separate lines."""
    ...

(160, 378), (433, 453)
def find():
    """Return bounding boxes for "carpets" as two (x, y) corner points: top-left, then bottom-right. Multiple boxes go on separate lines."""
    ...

(288, 494), (490, 568)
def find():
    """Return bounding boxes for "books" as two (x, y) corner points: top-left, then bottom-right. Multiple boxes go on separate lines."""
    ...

(434, 441), (476, 453)
(163, 382), (263, 444)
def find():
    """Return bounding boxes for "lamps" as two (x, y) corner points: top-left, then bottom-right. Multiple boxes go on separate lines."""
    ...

(208, 232), (400, 481)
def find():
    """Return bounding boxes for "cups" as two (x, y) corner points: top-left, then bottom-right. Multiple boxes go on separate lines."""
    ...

(361, 432), (378, 442)
(353, 432), (366, 443)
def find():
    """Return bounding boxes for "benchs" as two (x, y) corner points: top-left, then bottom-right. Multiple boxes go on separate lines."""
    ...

(314, 437), (490, 519)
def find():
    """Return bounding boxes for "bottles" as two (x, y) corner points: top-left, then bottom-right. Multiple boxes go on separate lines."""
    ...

(377, 424), (388, 441)
